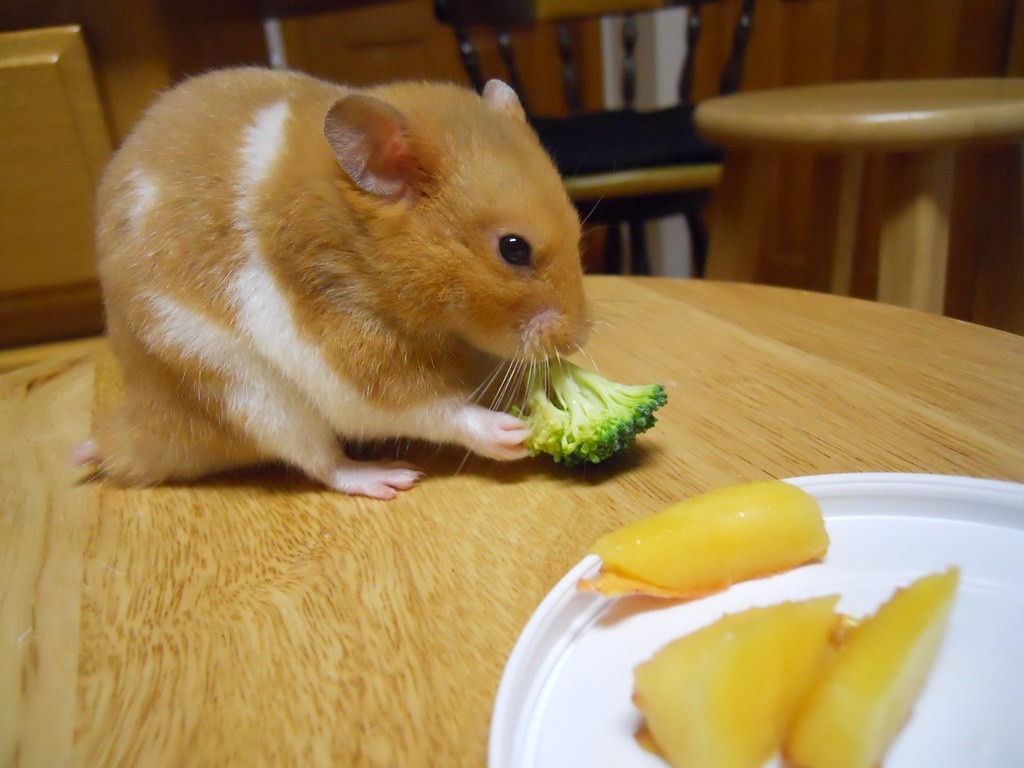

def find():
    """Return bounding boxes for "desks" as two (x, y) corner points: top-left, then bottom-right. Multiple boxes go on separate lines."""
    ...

(695, 78), (1024, 321)
(1, 274), (1024, 768)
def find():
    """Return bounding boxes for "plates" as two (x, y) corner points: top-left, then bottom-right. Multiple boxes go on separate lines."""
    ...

(487, 472), (1024, 768)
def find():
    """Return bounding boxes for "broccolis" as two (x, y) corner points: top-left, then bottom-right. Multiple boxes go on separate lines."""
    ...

(508, 356), (667, 466)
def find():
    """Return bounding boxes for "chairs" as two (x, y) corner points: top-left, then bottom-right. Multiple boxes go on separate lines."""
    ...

(431, 0), (755, 279)
